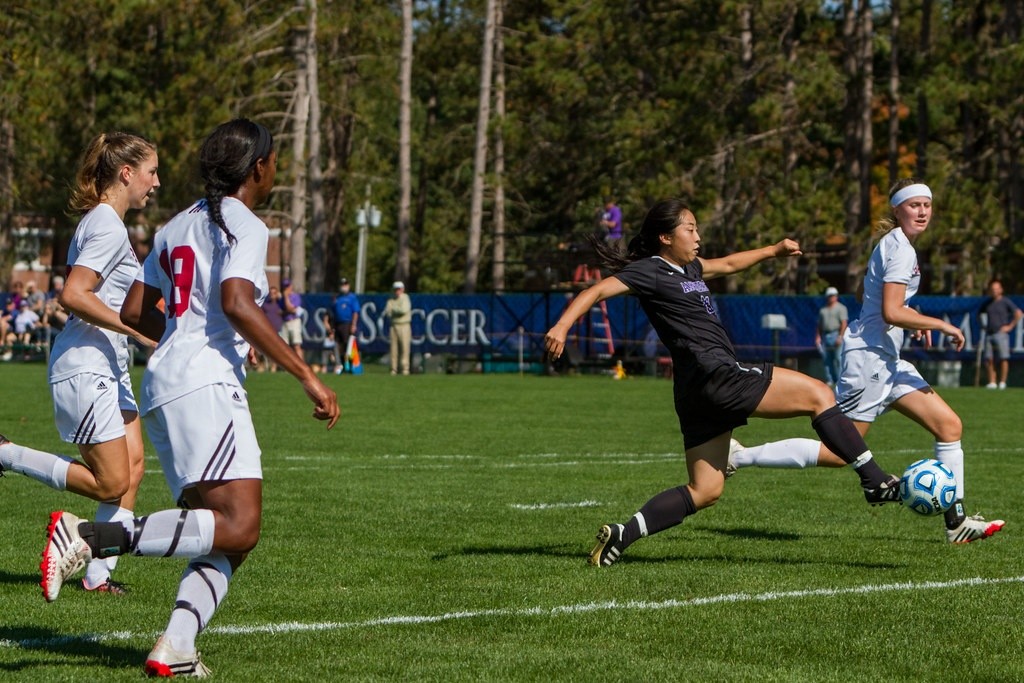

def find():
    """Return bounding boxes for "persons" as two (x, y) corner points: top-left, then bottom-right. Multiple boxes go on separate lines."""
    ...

(725, 177), (1005, 544)
(815, 287), (848, 385)
(323, 277), (360, 374)
(41, 119), (344, 679)
(381, 281), (412, 376)
(544, 198), (906, 570)
(978, 280), (1022, 389)
(261, 278), (303, 374)
(593, 196), (622, 243)
(0, 131), (161, 595)
(0, 275), (70, 361)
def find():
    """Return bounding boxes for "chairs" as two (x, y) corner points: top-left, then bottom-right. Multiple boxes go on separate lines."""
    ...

(0, 293), (67, 359)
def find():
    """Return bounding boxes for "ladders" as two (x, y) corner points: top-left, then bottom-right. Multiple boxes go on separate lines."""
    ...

(574, 262), (613, 360)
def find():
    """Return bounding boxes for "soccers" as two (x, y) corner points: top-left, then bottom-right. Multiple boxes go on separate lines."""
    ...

(898, 457), (960, 516)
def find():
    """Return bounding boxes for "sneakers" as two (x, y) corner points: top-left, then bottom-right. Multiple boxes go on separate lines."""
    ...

(725, 438), (744, 477)
(39, 510), (95, 601)
(146, 635), (212, 677)
(947, 512), (1005, 543)
(864, 474), (905, 506)
(80, 576), (133, 594)
(588, 524), (627, 569)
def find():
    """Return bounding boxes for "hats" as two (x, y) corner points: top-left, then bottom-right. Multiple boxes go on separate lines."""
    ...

(339, 277), (349, 283)
(825, 287), (839, 295)
(393, 281), (404, 288)
